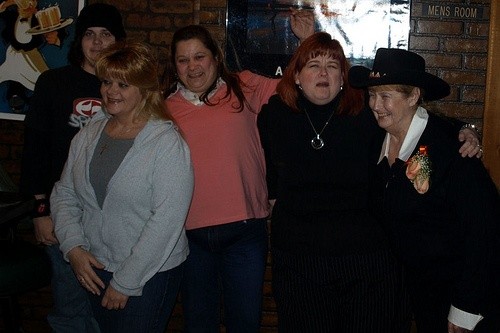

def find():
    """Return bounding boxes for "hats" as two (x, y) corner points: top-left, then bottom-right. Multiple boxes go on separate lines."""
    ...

(76, 8), (122, 43)
(348, 48), (447, 99)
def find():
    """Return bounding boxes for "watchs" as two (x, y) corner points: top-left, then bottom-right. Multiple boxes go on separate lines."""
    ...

(462, 123), (479, 132)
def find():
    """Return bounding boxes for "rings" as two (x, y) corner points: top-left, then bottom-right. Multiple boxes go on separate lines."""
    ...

(79, 277), (84, 281)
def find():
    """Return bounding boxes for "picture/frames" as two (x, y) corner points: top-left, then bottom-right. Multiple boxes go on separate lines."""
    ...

(224, 0), (412, 78)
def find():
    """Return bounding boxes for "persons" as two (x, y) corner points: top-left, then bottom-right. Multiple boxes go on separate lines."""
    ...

(19, 2), (500, 333)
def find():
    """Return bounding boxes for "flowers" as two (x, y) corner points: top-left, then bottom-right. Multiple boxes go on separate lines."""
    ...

(403, 146), (430, 194)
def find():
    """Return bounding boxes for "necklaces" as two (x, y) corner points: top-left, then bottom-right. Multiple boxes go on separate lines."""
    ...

(100, 116), (130, 154)
(301, 96), (338, 150)
